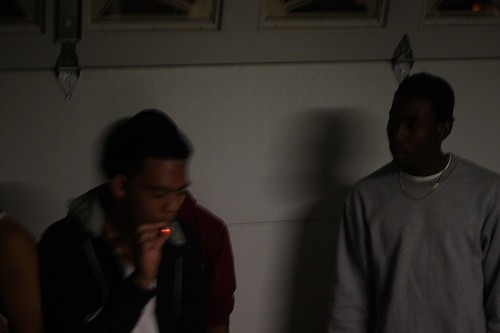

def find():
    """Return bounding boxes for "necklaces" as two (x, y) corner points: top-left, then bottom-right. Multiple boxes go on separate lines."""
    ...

(399, 164), (444, 199)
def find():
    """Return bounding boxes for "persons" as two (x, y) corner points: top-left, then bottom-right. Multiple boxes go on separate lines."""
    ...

(333, 72), (500, 333)
(37, 108), (237, 333)
(0, 209), (41, 333)
